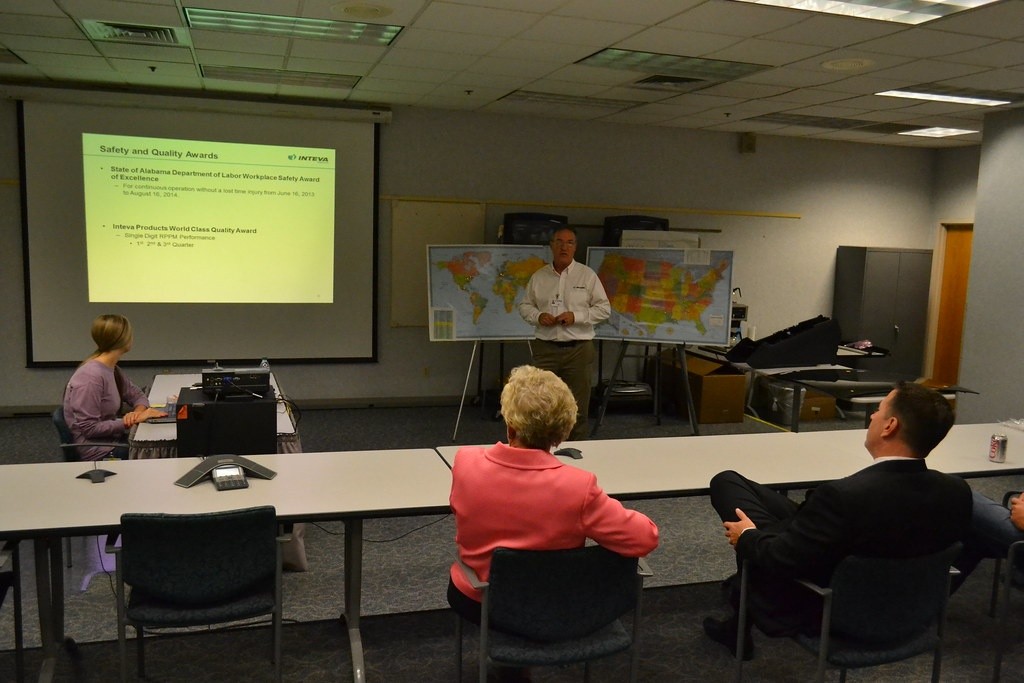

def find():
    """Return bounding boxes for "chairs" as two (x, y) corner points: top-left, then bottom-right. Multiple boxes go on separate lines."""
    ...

(453, 544), (655, 683)
(53, 406), (130, 462)
(988, 490), (1024, 683)
(104, 506), (285, 683)
(732, 539), (964, 683)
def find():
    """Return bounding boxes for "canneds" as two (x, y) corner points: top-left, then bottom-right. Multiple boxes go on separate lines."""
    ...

(990, 433), (1009, 464)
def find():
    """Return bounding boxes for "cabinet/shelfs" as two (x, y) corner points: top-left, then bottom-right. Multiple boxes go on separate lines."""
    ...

(832, 246), (933, 379)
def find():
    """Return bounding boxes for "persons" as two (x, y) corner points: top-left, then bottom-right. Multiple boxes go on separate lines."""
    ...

(63, 313), (169, 461)
(702, 381), (973, 660)
(972, 490), (1024, 559)
(518, 224), (612, 441)
(447, 365), (659, 683)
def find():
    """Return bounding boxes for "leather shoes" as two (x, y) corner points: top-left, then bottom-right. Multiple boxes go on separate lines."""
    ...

(702, 616), (755, 662)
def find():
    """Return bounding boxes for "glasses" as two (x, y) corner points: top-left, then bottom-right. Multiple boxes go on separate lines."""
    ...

(551, 238), (576, 247)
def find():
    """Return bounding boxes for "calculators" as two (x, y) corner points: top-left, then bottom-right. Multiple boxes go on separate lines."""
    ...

(212, 465), (250, 491)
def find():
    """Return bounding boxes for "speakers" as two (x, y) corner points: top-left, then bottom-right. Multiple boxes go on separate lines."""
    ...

(740, 136), (757, 154)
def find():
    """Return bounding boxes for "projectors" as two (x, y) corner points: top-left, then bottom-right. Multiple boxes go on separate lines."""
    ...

(202, 368), (270, 394)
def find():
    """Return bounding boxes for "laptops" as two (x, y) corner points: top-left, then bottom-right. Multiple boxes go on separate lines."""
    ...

(147, 403), (177, 423)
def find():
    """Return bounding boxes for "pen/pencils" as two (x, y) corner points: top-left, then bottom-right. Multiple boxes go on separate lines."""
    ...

(557, 320), (566, 324)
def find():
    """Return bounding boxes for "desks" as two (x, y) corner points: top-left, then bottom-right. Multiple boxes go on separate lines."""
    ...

(685, 348), (956, 429)
(0, 420), (1024, 683)
(128, 372), (310, 571)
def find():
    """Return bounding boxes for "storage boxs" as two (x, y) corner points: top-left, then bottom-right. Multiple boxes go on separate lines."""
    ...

(645, 347), (748, 424)
